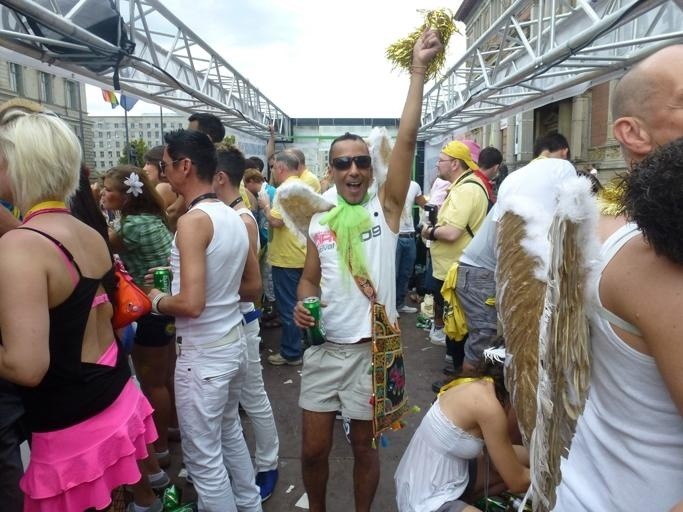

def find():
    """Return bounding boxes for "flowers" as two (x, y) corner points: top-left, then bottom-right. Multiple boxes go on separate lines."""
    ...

(124, 172), (144, 197)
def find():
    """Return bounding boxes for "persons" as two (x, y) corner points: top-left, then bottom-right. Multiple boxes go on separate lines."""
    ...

(548, 42), (683, 512)
(0, 115), (597, 512)
(292, 22), (445, 512)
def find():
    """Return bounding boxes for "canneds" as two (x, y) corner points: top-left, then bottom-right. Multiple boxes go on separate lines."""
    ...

(303, 295), (328, 346)
(153, 267), (172, 296)
(478, 491), (531, 512)
(163, 484), (197, 512)
(416, 313), (432, 327)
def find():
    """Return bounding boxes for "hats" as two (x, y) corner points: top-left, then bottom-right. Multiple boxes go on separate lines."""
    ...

(440, 139), (480, 171)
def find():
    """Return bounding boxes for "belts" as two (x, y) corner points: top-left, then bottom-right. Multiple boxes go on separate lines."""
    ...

(399, 232), (416, 238)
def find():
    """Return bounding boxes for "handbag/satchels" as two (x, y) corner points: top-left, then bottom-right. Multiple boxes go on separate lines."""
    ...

(111, 272), (153, 329)
(369, 303), (421, 450)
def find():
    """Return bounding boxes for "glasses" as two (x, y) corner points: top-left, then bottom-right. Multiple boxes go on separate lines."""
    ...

(330, 156), (371, 171)
(159, 158), (194, 174)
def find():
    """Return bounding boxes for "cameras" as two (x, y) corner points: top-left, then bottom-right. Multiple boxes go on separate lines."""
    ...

(415, 203), (438, 233)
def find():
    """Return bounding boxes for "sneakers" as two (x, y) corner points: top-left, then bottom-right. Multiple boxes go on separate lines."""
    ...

(123, 428), (180, 512)
(268, 353), (303, 366)
(430, 334), (446, 346)
(255, 469), (278, 503)
(432, 377), (452, 393)
(443, 365), (455, 377)
(398, 305), (417, 314)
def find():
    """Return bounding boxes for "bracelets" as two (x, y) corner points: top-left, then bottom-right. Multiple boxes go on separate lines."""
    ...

(412, 65), (428, 70)
